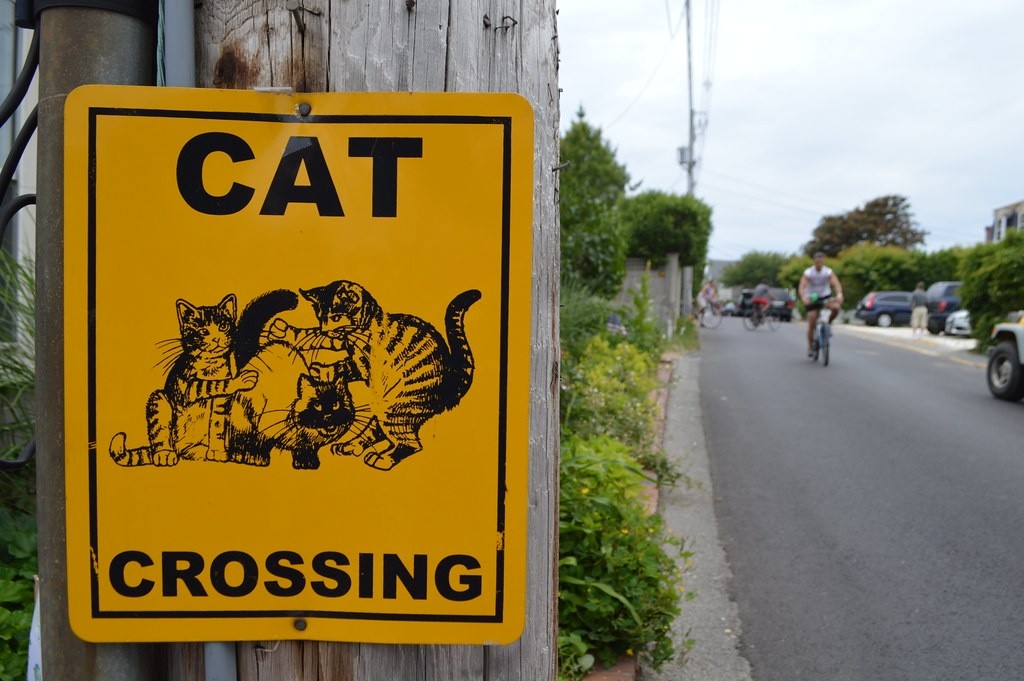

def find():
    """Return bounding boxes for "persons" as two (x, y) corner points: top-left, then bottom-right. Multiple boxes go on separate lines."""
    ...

(798, 252), (843, 357)
(909, 281), (928, 337)
(695, 279), (716, 327)
(752, 279), (770, 318)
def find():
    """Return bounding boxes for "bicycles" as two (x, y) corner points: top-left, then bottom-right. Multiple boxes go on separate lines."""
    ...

(806, 293), (835, 367)
(743, 297), (781, 332)
(699, 297), (722, 330)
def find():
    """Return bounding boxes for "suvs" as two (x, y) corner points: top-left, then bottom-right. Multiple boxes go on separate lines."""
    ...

(926, 281), (963, 335)
(987, 316), (1024, 402)
(855, 291), (913, 327)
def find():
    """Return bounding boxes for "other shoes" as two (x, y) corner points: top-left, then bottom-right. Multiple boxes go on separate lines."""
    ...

(808, 347), (816, 356)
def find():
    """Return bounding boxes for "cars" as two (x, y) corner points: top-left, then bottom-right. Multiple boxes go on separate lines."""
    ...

(945, 309), (974, 338)
(738, 287), (792, 322)
